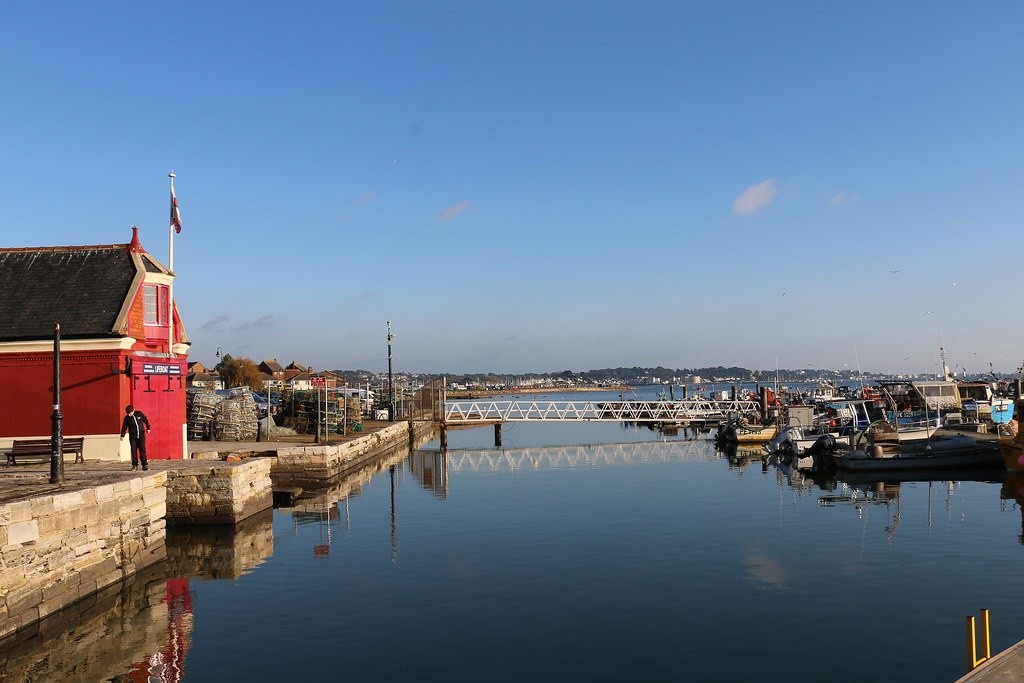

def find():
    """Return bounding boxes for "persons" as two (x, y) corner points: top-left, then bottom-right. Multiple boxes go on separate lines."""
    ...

(120, 405), (151, 471)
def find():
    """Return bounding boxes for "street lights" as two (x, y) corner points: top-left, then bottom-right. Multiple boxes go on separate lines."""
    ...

(216, 346), (223, 390)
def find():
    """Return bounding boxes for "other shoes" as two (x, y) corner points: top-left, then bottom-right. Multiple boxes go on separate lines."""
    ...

(131, 466), (139, 471)
(142, 466), (150, 471)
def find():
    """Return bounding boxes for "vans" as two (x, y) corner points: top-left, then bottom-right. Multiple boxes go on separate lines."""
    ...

(216, 387), (390, 426)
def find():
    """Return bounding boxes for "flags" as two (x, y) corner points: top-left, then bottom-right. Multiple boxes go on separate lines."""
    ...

(171, 186), (181, 234)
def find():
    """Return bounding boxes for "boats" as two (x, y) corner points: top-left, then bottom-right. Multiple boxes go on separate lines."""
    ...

(596, 325), (1024, 473)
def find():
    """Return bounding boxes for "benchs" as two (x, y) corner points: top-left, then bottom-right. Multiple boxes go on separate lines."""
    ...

(5, 437), (85, 468)
